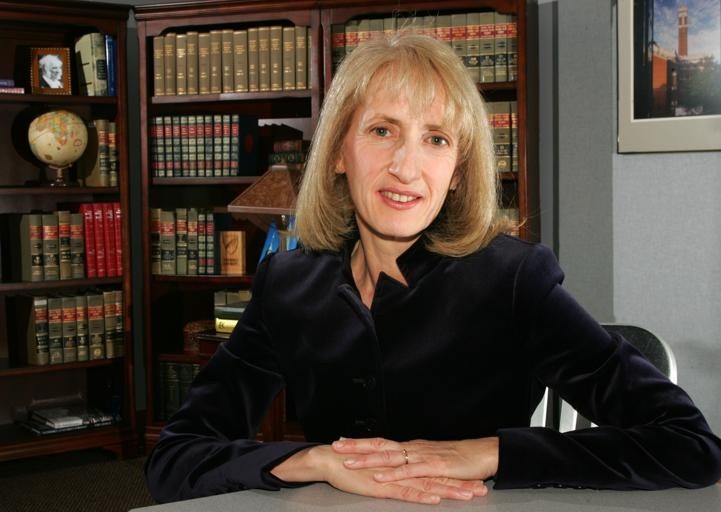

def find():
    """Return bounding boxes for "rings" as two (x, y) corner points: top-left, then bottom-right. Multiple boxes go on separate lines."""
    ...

(400, 446), (413, 466)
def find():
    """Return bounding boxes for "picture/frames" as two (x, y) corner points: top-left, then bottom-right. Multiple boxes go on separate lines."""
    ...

(612, 1), (721, 152)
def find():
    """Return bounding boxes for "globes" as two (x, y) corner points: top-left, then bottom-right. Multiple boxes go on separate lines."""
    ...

(27, 109), (88, 189)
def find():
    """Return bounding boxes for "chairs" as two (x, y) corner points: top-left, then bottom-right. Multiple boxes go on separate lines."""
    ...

(544, 325), (678, 436)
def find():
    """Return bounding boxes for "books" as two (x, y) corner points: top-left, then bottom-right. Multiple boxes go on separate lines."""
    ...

(149, 208), (246, 275)
(74, 31), (116, 96)
(0, 76), (25, 95)
(330, 12), (523, 85)
(150, 114), (307, 177)
(482, 96), (524, 242)
(150, 284), (253, 429)
(0, 203), (126, 364)
(87, 119), (119, 187)
(153, 25), (308, 96)
(13, 396), (117, 439)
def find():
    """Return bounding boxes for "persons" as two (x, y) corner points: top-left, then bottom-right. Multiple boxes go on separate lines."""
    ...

(38, 54), (64, 89)
(141, 31), (721, 505)
